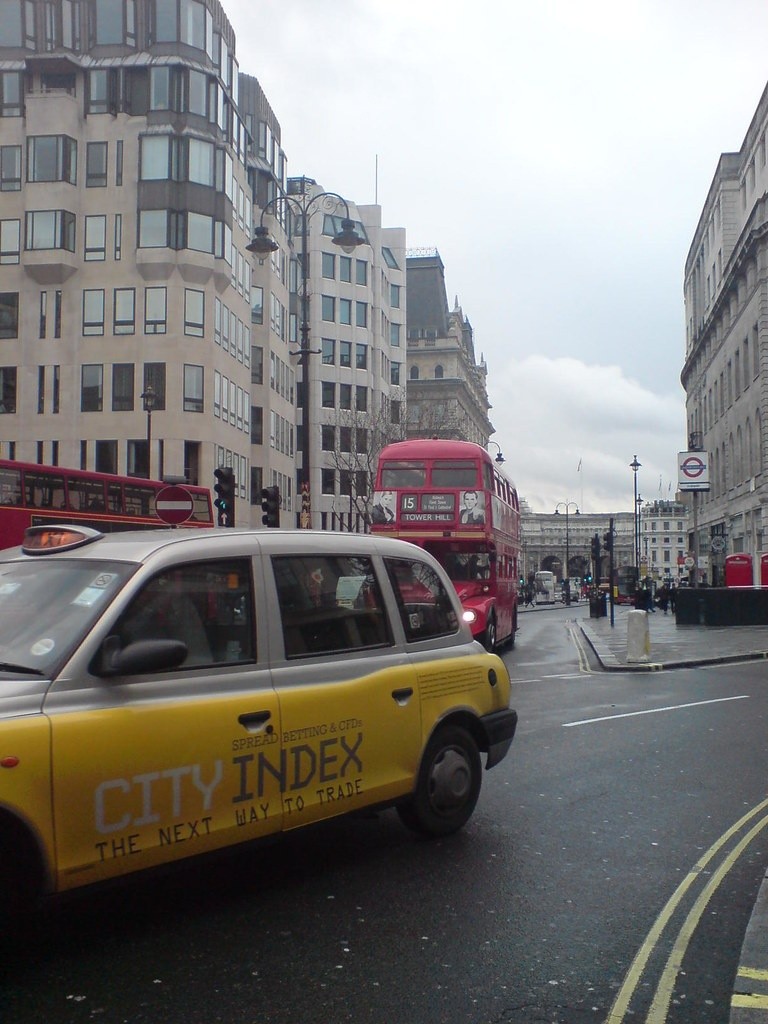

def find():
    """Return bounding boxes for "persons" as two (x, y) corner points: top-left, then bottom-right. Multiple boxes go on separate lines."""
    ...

(525, 591), (535, 609)
(644, 583), (677, 615)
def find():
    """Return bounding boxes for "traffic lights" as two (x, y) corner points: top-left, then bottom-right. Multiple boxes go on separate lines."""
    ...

(213, 467), (236, 528)
(604, 531), (611, 552)
(519, 578), (524, 587)
(590, 537), (597, 561)
(587, 574), (593, 586)
(260, 486), (281, 528)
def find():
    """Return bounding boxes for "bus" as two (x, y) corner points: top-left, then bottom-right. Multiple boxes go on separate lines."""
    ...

(370, 440), (521, 652)
(536, 571), (555, 605)
(0, 460), (216, 551)
(614, 566), (637, 604)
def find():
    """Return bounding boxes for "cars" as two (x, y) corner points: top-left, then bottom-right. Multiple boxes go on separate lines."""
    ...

(0, 524), (517, 978)
(554, 590), (578, 603)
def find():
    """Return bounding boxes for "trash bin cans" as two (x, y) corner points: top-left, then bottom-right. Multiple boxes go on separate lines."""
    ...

(635, 589), (650, 610)
(589, 592), (607, 618)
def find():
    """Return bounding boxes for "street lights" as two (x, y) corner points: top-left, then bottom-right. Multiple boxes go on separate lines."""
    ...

(629, 454), (643, 591)
(553, 501), (581, 605)
(140, 386), (159, 476)
(635, 493), (644, 578)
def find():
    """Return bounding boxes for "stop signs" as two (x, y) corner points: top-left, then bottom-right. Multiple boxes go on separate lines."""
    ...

(153, 485), (196, 525)
